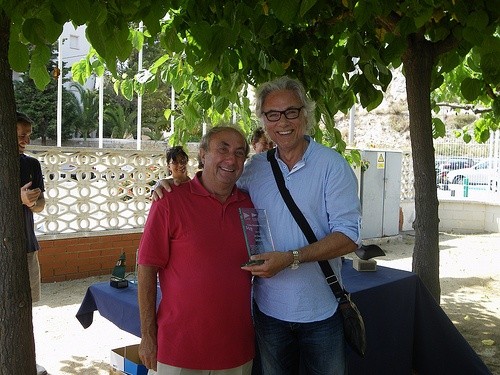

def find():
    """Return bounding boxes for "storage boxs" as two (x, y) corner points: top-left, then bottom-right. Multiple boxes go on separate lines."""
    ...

(110, 344), (149, 375)
(353, 258), (377, 271)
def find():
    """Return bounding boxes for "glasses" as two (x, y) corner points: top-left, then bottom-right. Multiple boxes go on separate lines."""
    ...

(168, 159), (188, 165)
(262, 104), (307, 122)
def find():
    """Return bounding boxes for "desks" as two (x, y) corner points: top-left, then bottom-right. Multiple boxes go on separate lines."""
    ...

(76, 260), (492, 375)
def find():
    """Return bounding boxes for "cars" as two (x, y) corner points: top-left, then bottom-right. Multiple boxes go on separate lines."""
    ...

(435, 155), (498, 183)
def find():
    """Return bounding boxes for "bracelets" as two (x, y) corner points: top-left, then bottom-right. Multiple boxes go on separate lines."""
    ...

(26, 203), (36, 209)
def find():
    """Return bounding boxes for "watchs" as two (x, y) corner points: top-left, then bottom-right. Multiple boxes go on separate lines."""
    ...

(289, 250), (299, 271)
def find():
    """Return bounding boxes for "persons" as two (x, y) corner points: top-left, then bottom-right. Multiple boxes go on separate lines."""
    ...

(136, 125), (256, 375)
(152, 78), (361, 375)
(252, 128), (273, 153)
(151, 146), (190, 196)
(13, 112), (46, 303)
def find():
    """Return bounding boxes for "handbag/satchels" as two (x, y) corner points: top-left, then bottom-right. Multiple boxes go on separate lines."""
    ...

(340, 295), (367, 355)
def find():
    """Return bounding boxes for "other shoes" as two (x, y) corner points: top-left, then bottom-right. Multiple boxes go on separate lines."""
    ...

(35, 364), (48, 374)
